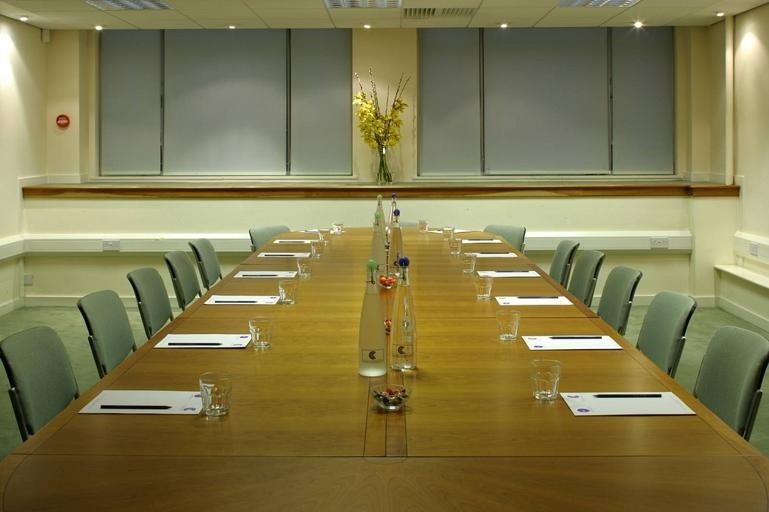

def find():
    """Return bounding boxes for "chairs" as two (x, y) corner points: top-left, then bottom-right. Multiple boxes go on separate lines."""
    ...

(163, 249), (202, 313)
(567, 249), (606, 308)
(126, 267), (174, 339)
(595, 265), (642, 335)
(75, 290), (138, 381)
(248, 225), (290, 257)
(188, 237), (223, 291)
(635, 290), (699, 381)
(482, 224), (529, 259)
(543, 239), (580, 293)
(1, 326), (81, 445)
(691, 325), (769, 442)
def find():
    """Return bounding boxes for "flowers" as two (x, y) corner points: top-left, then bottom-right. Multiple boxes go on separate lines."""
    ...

(351, 64), (410, 149)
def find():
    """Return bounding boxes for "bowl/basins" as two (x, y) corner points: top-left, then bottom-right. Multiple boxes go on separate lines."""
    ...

(370, 384), (413, 411)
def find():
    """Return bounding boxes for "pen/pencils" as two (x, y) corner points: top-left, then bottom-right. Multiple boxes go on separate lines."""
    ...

(593, 392), (663, 398)
(166, 341), (222, 346)
(517, 295), (558, 300)
(481, 250), (509, 256)
(466, 237), (494, 241)
(494, 268), (529, 274)
(99, 403), (173, 410)
(265, 252), (295, 256)
(277, 240), (306, 244)
(214, 299), (256, 304)
(549, 336), (603, 341)
(241, 273), (279, 279)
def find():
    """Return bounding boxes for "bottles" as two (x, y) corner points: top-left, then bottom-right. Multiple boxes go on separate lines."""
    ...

(358, 260), (388, 377)
(390, 257), (417, 370)
(387, 193), (398, 241)
(370, 212), (386, 272)
(388, 209), (403, 273)
(376, 195), (385, 228)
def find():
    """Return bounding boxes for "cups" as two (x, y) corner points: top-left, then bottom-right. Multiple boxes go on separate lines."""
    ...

(248, 318), (274, 350)
(297, 258), (312, 279)
(462, 254), (476, 273)
(199, 371), (232, 416)
(278, 278), (298, 305)
(311, 241), (326, 258)
(474, 276), (492, 300)
(495, 310), (520, 341)
(448, 238), (462, 255)
(334, 223), (343, 236)
(529, 359), (560, 400)
(419, 220), (429, 233)
(319, 229), (330, 244)
(442, 226), (454, 241)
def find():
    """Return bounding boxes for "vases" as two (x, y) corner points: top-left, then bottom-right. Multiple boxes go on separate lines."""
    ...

(373, 145), (393, 187)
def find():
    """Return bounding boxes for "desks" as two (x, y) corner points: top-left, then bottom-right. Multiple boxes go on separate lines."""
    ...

(0, 229), (769, 512)
